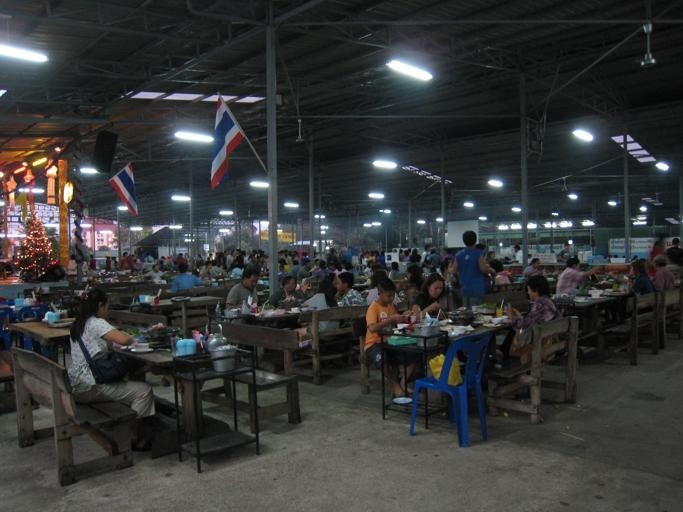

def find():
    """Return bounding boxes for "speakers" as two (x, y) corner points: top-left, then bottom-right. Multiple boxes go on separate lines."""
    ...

(90, 130), (118, 172)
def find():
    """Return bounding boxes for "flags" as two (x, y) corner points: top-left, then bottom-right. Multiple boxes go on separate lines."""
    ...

(108, 162), (140, 218)
(210, 90), (248, 188)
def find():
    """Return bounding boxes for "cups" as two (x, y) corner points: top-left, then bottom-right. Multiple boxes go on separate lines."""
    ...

(495, 307), (503, 318)
(134, 343), (148, 351)
(591, 290), (600, 299)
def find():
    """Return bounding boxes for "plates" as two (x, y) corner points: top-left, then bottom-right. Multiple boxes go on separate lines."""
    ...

(49, 320), (75, 328)
(170, 295), (191, 302)
(130, 348), (154, 352)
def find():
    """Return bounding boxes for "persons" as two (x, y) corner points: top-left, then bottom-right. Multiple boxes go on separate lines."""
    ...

(363, 278), (423, 407)
(68, 287), (157, 450)
(412, 272), (468, 376)
(62, 227), (683, 367)
(496, 275), (562, 387)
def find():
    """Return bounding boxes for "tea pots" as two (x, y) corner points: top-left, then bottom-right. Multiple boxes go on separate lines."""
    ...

(199, 322), (226, 353)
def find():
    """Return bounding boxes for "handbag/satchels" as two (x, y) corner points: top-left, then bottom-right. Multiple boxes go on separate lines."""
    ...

(429, 354), (463, 387)
(89, 354), (130, 384)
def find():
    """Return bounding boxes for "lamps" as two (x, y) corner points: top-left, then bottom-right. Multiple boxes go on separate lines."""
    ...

(639, 24), (659, 68)
(562, 178), (568, 192)
(296, 118), (304, 142)
(61, 182), (74, 205)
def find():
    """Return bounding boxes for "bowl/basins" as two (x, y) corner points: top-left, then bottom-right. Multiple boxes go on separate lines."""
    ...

(414, 326), (440, 347)
(452, 326), (465, 334)
